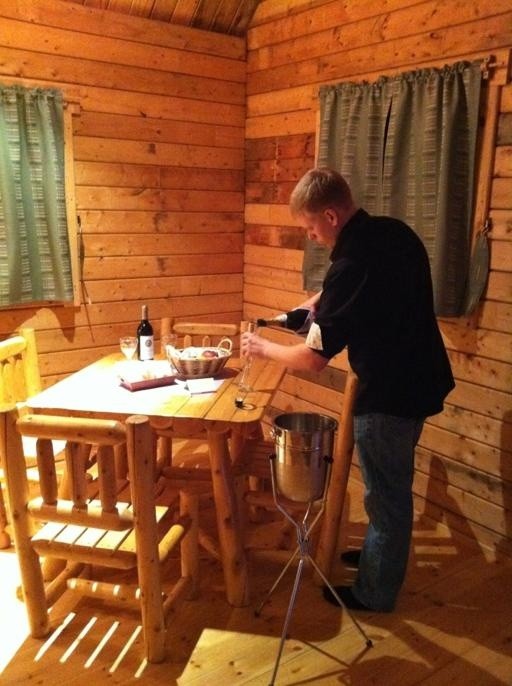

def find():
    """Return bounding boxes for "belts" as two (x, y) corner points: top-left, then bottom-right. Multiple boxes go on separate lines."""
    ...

(139, 305), (154, 361)
(162, 334), (175, 361)
(119, 337), (138, 360)
(231, 341), (256, 390)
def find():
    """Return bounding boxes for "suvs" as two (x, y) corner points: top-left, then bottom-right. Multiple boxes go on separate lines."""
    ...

(270, 411), (338, 501)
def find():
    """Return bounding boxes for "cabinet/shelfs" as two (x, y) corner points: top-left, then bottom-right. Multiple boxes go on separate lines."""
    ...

(257, 310), (313, 333)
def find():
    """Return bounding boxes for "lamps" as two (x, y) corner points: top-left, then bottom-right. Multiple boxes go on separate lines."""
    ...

(0, 327), (65, 548)
(232, 372), (359, 586)
(0, 405), (201, 663)
(159, 317), (251, 466)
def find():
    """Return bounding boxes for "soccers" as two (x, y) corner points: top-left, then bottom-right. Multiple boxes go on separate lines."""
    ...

(203, 350), (218, 358)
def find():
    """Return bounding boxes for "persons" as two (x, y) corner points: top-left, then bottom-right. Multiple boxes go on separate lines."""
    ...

(241, 166), (455, 612)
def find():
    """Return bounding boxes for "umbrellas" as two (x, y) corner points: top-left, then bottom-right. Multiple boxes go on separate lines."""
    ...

(339, 545), (362, 568)
(321, 583), (399, 612)
